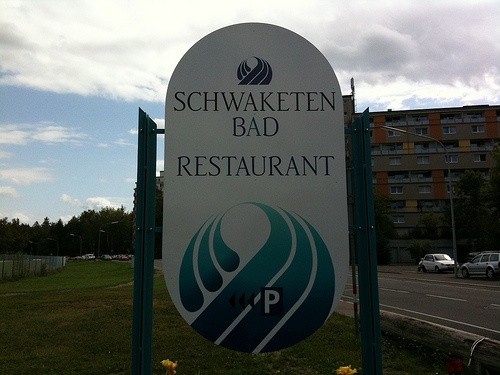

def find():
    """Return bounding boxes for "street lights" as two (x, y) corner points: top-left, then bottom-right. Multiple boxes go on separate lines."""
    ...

(48, 238), (59, 256)
(70, 234), (82, 257)
(379, 125), (458, 276)
(98, 222), (118, 258)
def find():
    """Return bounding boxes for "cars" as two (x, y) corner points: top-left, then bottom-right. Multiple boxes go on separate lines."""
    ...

(102, 253), (133, 261)
(75, 254), (96, 260)
(459, 252), (500, 279)
(417, 254), (459, 272)
